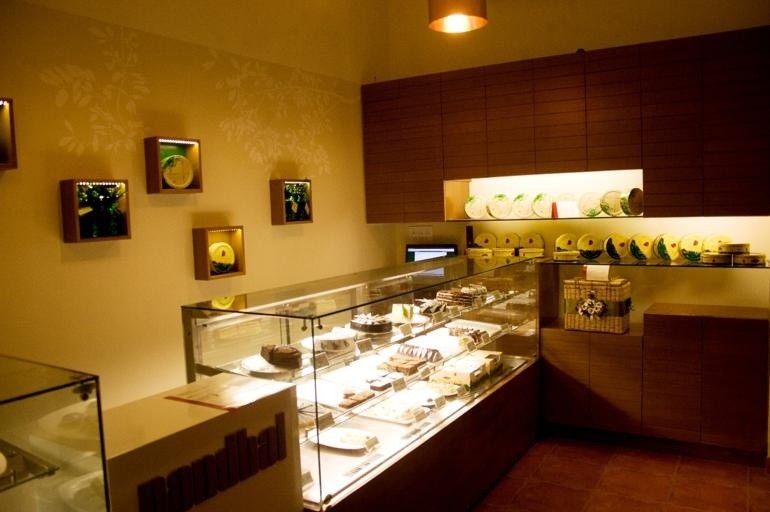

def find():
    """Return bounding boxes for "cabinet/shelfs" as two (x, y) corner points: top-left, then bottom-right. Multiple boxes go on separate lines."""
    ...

(643, 25), (769, 224)
(1, 96), (17, 169)
(61, 179), (131, 242)
(361, 66), (470, 224)
(0, 354), (112, 512)
(192, 225), (247, 280)
(178, 253), (544, 512)
(643, 302), (770, 456)
(145, 136), (203, 194)
(269, 179), (313, 226)
(471, 42), (643, 178)
(543, 318), (642, 434)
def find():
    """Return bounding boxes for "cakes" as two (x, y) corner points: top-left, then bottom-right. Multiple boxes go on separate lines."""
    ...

(437, 289), (472, 307)
(350, 312), (393, 345)
(460, 285), (487, 304)
(316, 329), (358, 353)
(419, 300), (447, 313)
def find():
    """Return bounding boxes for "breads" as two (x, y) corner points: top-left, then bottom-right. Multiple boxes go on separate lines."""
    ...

(392, 303), (413, 321)
(261, 345), (302, 369)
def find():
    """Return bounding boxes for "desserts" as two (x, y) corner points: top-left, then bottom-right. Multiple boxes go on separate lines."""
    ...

(375, 353), (427, 376)
(339, 390), (375, 409)
(448, 328), (487, 349)
(397, 344), (442, 363)
(365, 370), (403, 391)
(297, 400), (334, 430)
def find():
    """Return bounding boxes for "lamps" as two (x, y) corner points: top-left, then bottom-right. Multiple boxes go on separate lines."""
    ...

(427, 0), (490, 33)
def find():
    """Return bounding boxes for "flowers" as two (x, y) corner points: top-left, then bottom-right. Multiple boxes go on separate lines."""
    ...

(575, 290), (609, 320)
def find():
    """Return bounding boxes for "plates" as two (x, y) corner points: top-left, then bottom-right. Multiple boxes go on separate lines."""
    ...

(308, 427), (376, 450)
(345, 323), (396, 335)
(384, 312), (429, 326)
(300, 335), (356, 352)
(241, 354), (288, 374)
(58, 471), (107, 512)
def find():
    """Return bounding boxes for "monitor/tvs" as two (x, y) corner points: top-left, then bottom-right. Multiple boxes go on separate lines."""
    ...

(405, 243), (458, 263)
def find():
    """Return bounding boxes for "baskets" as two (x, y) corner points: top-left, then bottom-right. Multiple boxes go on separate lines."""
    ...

(562, 279), (631, 336)
